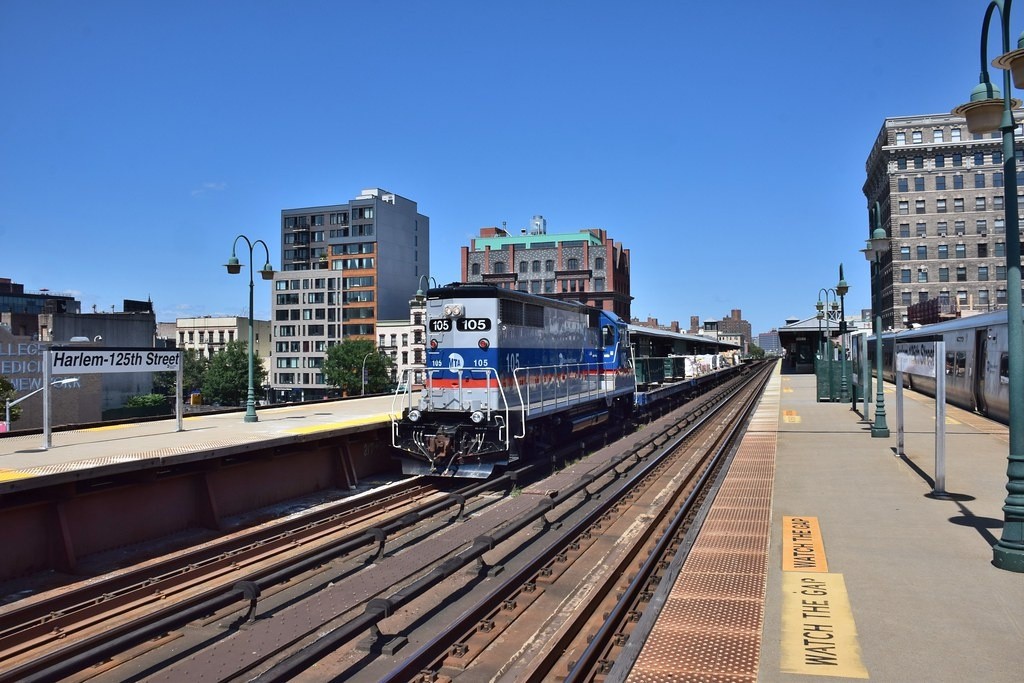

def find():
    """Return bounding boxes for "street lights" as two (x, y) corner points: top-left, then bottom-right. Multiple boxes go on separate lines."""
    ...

(816, 309), (826, 360)
(951, 0), (1024, 576)
(222, 234), (279, 422)
(813, 289), (840, 354)
(5, 377), (79, 433)
(361, 352), (374, 396)
(859, 202), (890, 438)
(834, 261), (852, 404)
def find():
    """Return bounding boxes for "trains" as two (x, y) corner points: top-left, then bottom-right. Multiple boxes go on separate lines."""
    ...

(866, 308), (1024, 425)
(389, 280), (746, 480)
